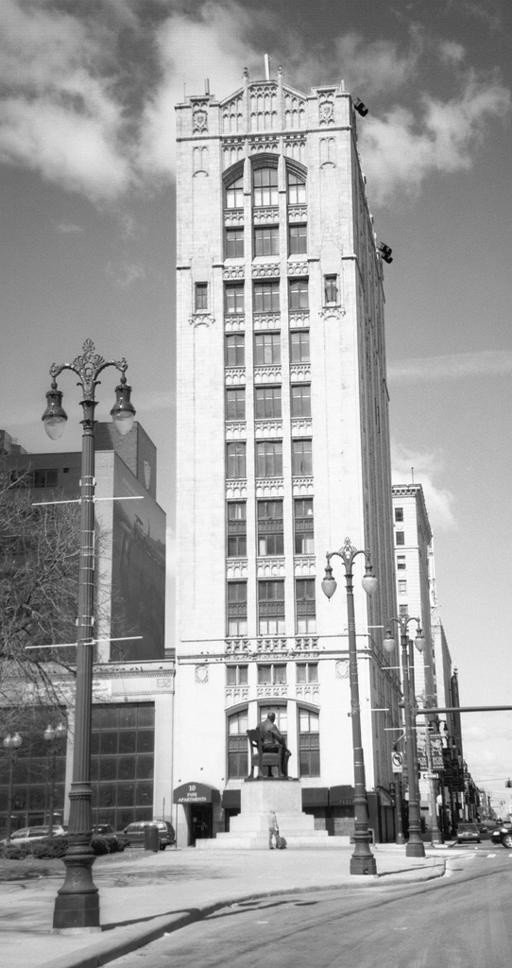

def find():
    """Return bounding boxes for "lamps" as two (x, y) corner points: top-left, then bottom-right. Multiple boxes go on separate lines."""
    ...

(353, 96), (369, 117)
(376, 241), (393, 263)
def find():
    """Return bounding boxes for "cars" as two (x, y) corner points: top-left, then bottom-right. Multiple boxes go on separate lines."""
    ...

(458, 823), (481, 844)
(0, 824), (68, 860)
(491, 821), (512, 850)
(475, 823), (489, 831)
(90, 821), (122, 851)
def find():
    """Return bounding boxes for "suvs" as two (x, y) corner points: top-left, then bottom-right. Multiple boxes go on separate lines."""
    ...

(114, 818), (175, 851)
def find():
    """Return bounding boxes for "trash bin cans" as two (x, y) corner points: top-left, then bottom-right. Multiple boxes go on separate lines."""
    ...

(143, 824), (160, 853)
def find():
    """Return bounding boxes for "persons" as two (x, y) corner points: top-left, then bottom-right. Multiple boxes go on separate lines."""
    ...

(266, 808), (282, 848)
(256, 712), (291, 776)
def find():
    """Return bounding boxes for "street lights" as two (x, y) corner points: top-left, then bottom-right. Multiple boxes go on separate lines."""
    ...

(321, 536), (378, 879)
(40, 335), (137, 925)
(3, 732), (24, 832)
(382, 611), (428, 856)
(41, 717), (66, 836)
(425, 713), (466, 840)
